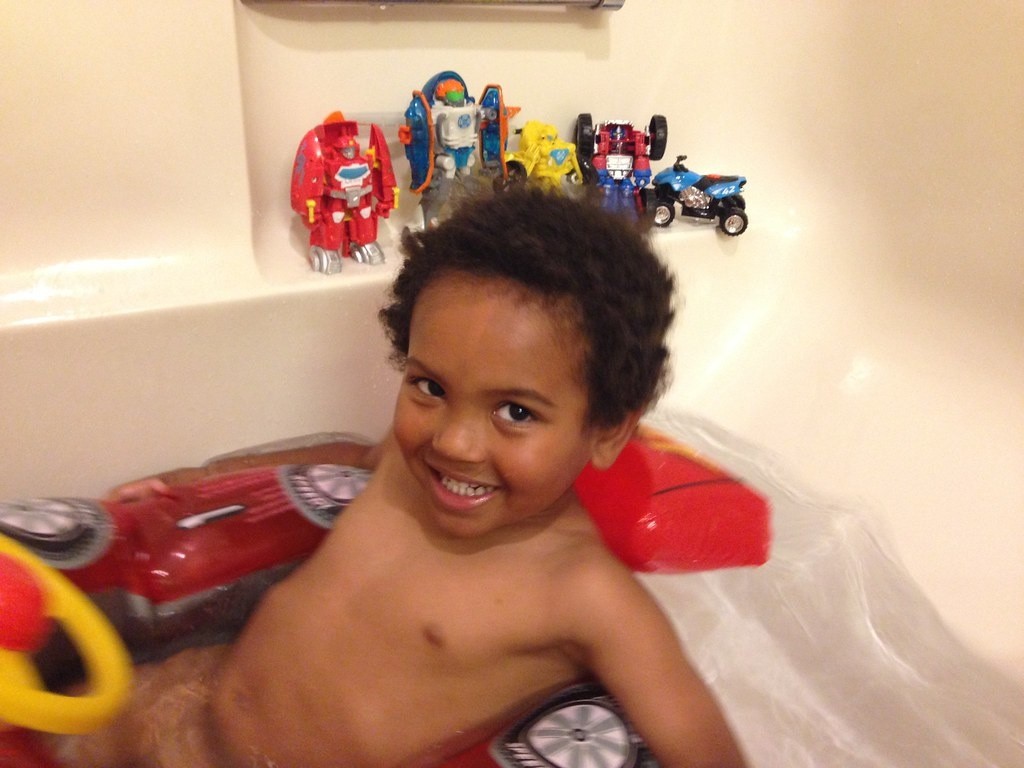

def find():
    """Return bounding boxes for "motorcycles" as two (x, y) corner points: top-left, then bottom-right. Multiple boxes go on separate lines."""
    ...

(652, 155), (749, 236)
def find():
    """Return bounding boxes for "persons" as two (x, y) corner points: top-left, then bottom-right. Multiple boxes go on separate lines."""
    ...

(45, 184), (747, 767)
(595, 122), (651, 234)
(399, 71), (521, 227)
(292, 112), (399, 275)
(508, 119), (583, 199)
